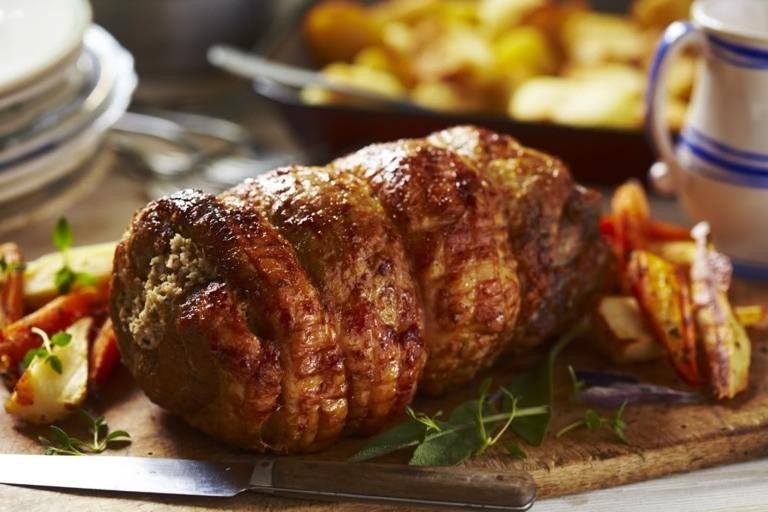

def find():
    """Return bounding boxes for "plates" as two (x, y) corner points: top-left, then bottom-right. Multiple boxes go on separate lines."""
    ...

(1, 1), (144, 233)
(204, 40), (645, 194)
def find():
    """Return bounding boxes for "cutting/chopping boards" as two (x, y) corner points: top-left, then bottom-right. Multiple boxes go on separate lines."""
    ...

(2, 275), (765, 504)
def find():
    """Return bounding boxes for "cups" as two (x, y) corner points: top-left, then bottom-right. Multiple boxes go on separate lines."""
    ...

(646, 1), (766, 281)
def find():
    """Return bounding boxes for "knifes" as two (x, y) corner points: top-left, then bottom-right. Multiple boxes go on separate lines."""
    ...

(0, 451), (537, 511)
